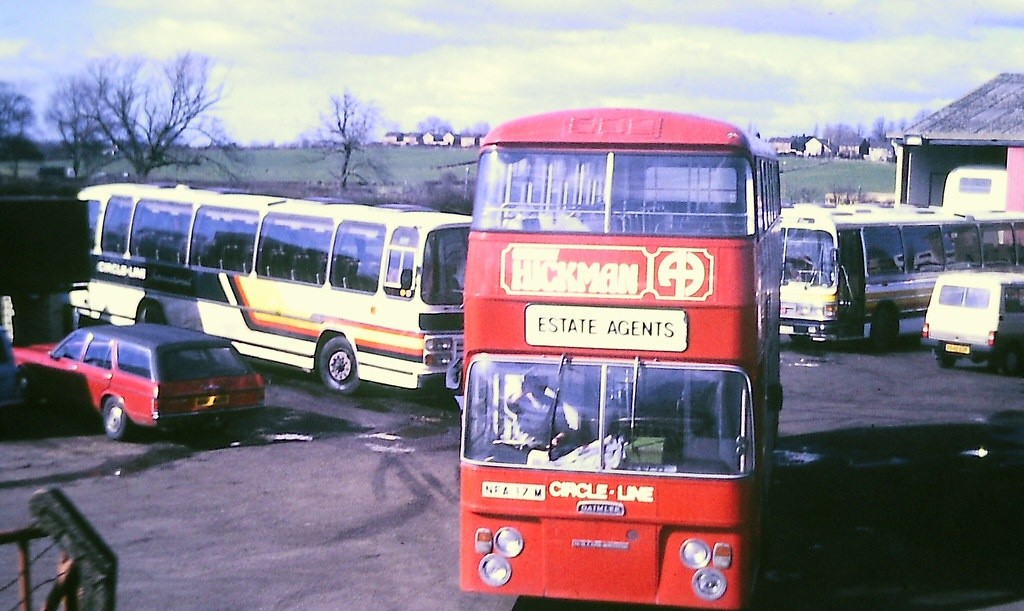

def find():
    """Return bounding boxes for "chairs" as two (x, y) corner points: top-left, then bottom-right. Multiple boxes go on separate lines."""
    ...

(497, 197), (739, 232)
(868, 249), (954, 274)
(101, 227), (378, 292)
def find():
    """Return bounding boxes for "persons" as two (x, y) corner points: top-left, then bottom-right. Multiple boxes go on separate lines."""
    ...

(506, 370), (569, 450)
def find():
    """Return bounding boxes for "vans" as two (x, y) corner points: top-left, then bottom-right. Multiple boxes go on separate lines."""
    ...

(920, 269), (1024, 373)
(941, 167), (1011, 215)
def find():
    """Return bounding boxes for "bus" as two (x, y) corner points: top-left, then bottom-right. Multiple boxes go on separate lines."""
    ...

(455, 109), (789, 611)
(774, 204), (1024, 353)
(70, 181), (474, 397)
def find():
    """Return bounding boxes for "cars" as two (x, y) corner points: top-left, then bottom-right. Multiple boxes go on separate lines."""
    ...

(0, 323), (27, 438)
(8, 322), (268, 438)
